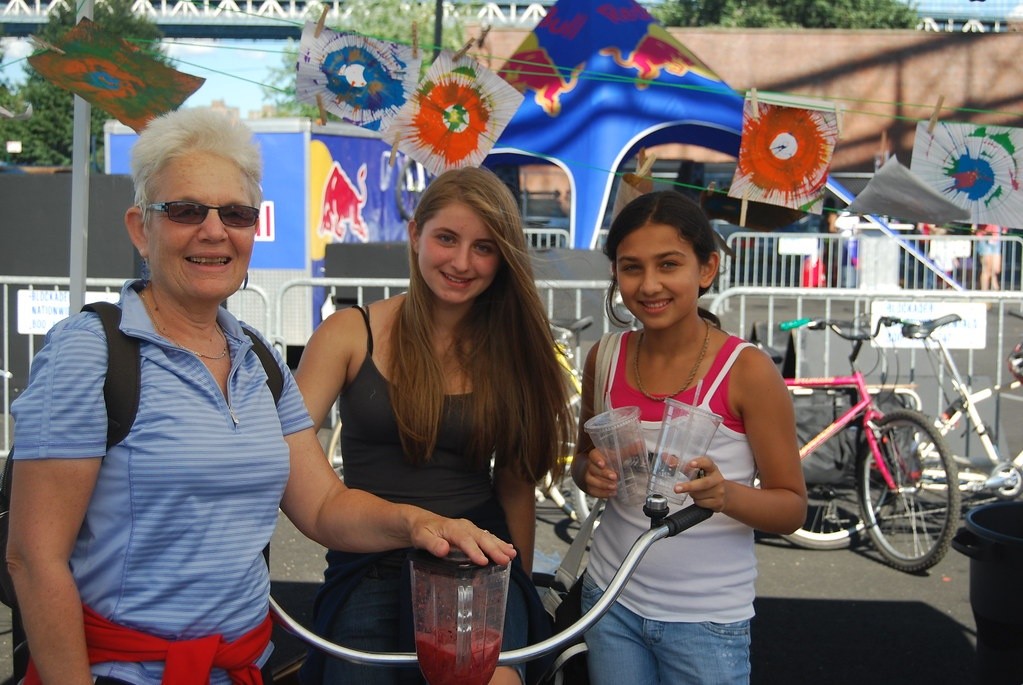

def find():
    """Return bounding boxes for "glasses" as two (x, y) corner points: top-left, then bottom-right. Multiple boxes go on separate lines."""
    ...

(144, 201), (261, 228)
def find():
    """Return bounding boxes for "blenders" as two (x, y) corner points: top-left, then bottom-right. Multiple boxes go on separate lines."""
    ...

(407, 546), (511, 685)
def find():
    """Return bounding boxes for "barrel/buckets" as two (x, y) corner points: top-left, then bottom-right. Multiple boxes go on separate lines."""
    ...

(950, 499), (1023, 685)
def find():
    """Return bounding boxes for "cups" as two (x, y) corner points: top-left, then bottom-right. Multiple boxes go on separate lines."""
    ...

(646, 397), (723, 505)
(584, 407), (650, 506)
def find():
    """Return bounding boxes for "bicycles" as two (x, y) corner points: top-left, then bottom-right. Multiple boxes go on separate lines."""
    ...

(778, 315), (1023, 549)
(533, 314), (605, 537)
(259, 492), (717, 685)
(776, 314), (964, 576)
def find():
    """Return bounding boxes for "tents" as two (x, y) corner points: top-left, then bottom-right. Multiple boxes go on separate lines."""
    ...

(483, 1), (963, 289)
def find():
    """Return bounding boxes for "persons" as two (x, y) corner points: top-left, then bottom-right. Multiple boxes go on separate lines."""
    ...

(572, 191), (807, 685)
(819, 212), (958, 288)
(972, 223), (1008, 289)
(6, 107), (517, 685)
(294, 167), (579, 685)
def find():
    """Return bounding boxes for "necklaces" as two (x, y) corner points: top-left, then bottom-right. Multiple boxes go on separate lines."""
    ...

(140, 290), (227, 359)
(634, 319), (709, 400)
